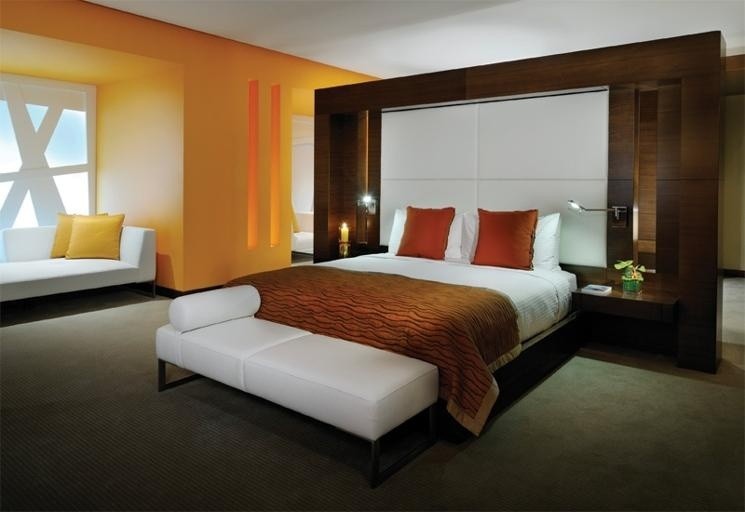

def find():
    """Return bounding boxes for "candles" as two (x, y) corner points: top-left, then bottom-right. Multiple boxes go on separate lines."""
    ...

(338, 224), (350, 254)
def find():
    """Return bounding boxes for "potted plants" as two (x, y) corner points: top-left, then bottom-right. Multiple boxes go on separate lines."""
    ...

(614, 259), (646, 294)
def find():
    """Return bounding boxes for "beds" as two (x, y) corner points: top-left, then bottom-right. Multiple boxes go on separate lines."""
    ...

(152, 250), (589, 488)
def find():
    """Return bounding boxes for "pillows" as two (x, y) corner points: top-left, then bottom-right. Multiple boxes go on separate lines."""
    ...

(165, 284), (262, 335)
(387, 205), (559, 272)
(49, 212), (126, 260)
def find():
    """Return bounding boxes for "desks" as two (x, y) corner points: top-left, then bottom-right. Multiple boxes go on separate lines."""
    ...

(571, 283), (678, 324)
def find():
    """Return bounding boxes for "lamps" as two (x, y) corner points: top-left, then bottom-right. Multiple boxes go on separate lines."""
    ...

(567, 199), (626, 230)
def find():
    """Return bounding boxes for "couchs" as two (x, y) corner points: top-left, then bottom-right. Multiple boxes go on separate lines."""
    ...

(1, 226), (159, 304)
